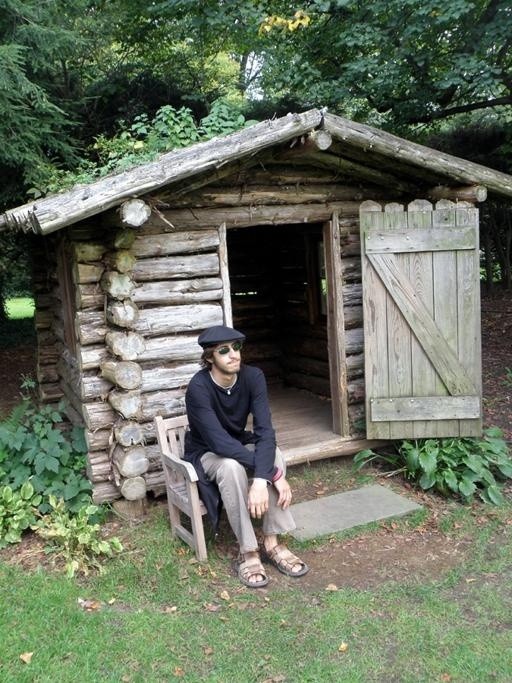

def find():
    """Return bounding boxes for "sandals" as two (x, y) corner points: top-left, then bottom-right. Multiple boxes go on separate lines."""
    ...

(261, 542), (308, 577)
(231, 549), (268, 587)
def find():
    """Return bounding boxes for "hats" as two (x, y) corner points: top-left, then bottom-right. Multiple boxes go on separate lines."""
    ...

(198, 325), (246, 345)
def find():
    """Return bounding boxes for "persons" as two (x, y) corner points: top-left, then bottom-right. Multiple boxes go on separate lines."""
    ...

(184, 323), (310, 586)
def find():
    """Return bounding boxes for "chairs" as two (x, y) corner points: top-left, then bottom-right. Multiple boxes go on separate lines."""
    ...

(153, 413), (247, 564)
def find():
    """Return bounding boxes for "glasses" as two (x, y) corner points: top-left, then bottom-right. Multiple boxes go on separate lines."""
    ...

(214, 343), (241, 354)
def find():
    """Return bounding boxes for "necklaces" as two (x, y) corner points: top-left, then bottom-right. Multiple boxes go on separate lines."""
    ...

(210, 371), (237, 395)
